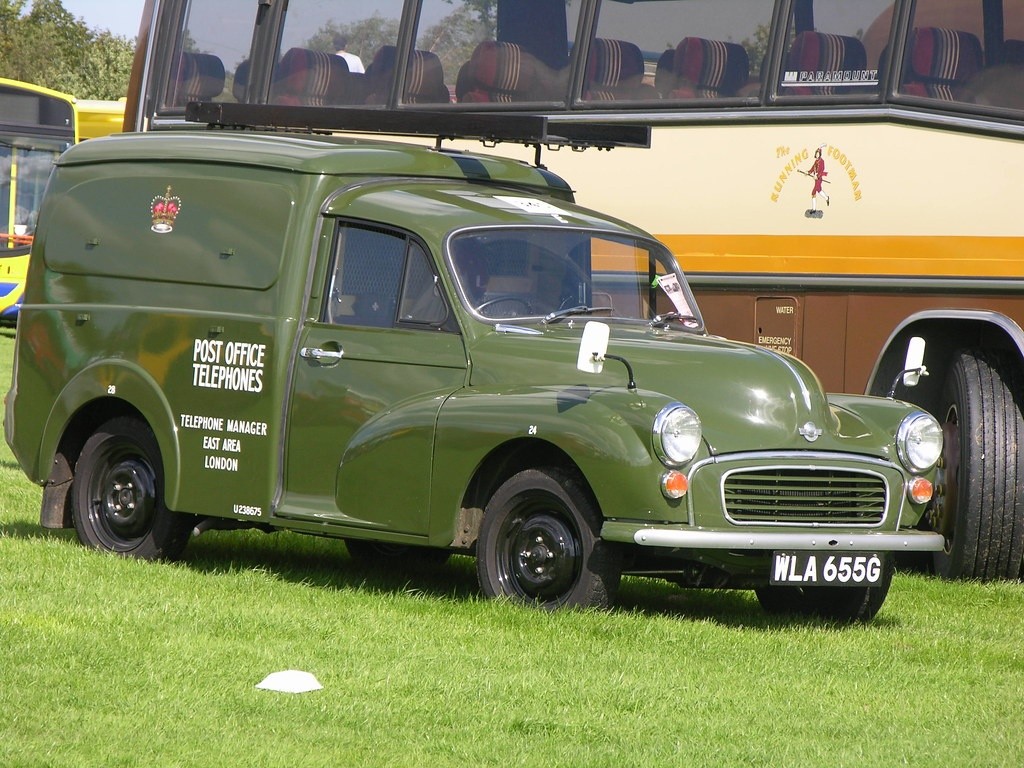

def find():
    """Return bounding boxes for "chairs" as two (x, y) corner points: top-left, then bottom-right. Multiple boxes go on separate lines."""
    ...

(411, 263), (475, 320)
(180, 26), (1023, 112)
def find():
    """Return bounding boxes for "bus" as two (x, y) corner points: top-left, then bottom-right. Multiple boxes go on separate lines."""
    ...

(1, 79), (128, 328)
(122, 1), (1024, 582)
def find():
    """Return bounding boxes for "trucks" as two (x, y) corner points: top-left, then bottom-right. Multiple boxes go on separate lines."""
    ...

(3, 101), (945, 625)
